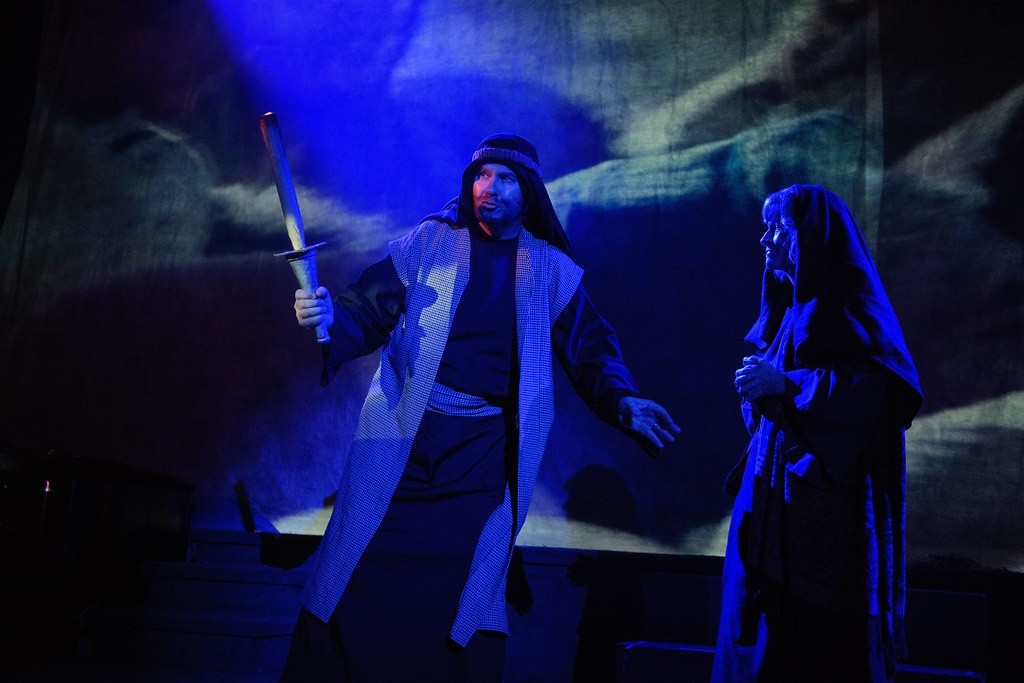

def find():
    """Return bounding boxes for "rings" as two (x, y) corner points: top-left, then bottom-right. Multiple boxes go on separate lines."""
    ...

(653, 423), (658, 430)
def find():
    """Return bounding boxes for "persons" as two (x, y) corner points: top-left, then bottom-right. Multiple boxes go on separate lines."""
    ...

(711, 184), (926, 683)
(292, 133), (682, 682)
